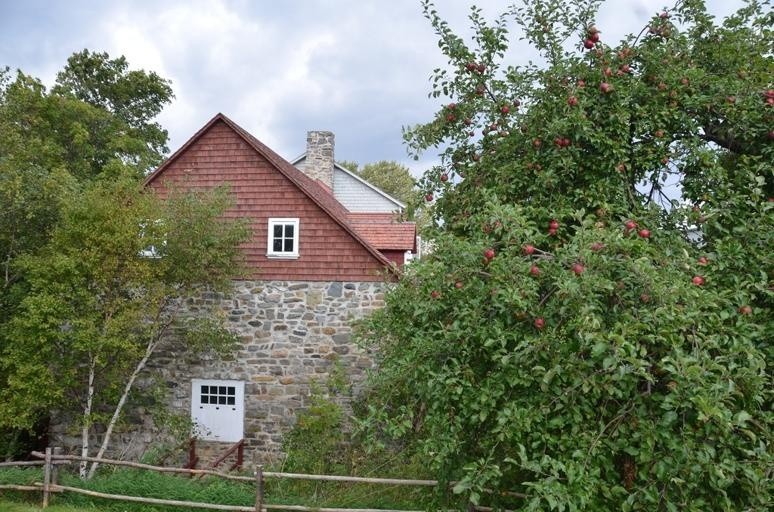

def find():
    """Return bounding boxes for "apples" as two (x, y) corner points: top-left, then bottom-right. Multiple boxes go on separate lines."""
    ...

(425, 14), (774, 327)
